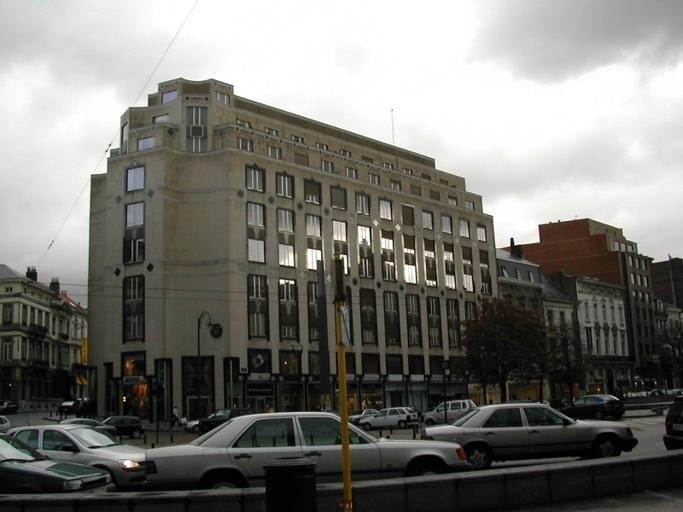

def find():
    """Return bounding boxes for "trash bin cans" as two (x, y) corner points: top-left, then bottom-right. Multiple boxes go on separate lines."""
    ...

(262, 456), (320, 512)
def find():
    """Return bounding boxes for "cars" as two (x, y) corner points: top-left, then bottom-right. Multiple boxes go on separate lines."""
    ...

(663, 395), (683, 449)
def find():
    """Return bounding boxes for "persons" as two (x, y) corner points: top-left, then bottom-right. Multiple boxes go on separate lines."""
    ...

(122, 398), (139, 418)
(361, 397), (371, 412)
(71, 397), (97, 421)
(321, 404), (330, 413)
(230, 402), (275, 418)
